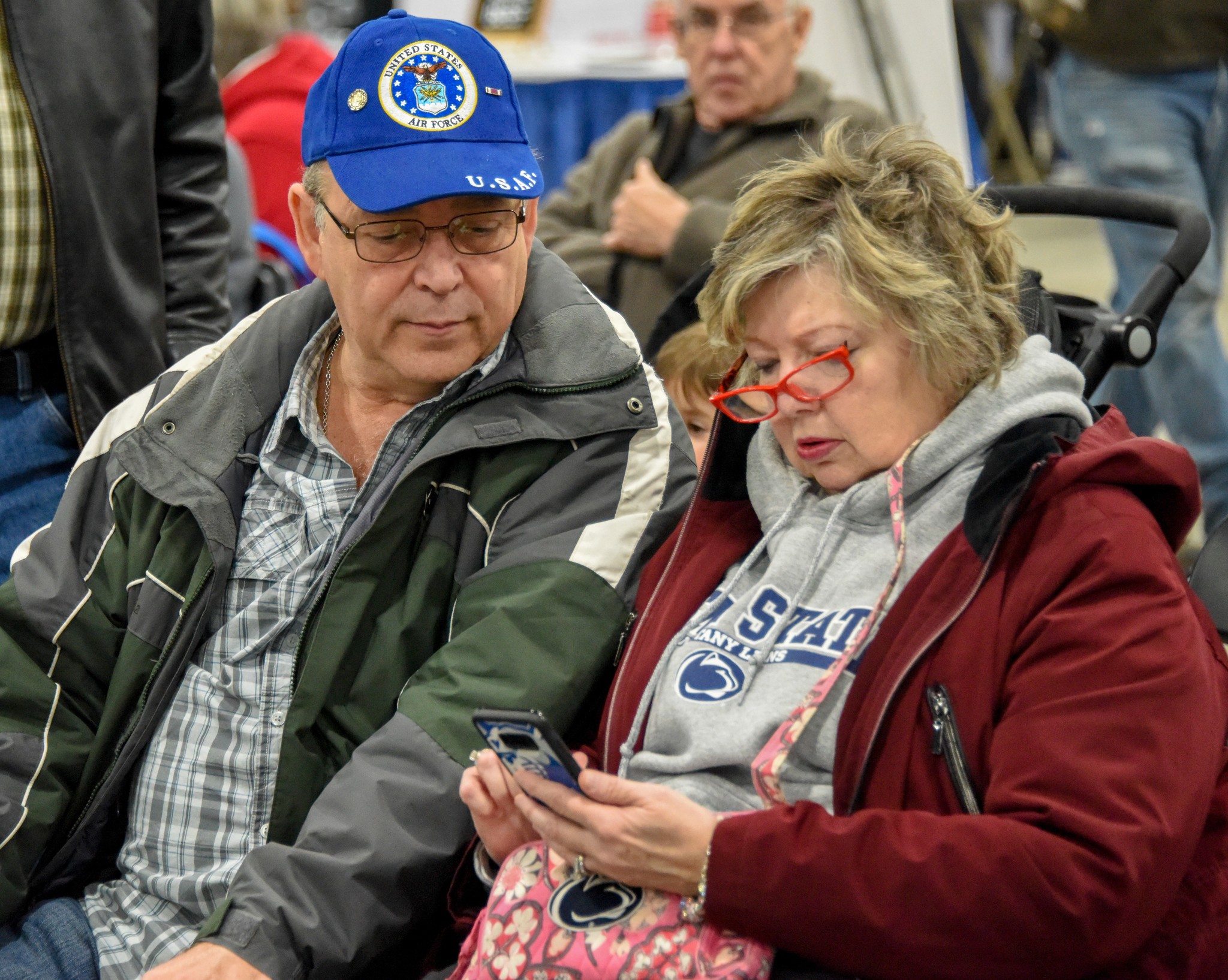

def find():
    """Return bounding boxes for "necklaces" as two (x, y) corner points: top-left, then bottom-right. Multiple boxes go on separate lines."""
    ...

(322, 329), (343, 434)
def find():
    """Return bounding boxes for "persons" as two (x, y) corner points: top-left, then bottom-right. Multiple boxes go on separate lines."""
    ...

(656, 326), (742, 469)
(1026, 0), (1228, 537)
(536, 1), (882, 363)
(0, 9), (697, 980)
(0, 0), (233, 588)
(448, 116), (1228, 980)
(210, 0), (335, 319)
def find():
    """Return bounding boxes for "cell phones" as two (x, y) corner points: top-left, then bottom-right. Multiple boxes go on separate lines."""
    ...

(473, 708), (585, 805)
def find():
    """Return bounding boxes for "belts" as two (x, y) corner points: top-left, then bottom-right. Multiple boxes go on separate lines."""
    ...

(0, 340), (67, 396)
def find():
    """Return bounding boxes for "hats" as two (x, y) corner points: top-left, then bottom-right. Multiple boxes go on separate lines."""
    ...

(301, 8), (544, 213)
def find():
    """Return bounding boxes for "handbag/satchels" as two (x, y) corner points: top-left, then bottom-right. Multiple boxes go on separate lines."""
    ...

(445, 837), (775, 980)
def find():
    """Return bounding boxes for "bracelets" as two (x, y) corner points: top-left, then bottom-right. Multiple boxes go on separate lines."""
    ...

(679, 844), (710, 923)
(478, 845), (496, 882)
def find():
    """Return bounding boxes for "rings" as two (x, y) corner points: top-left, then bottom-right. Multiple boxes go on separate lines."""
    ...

(576, 855), (589, 878)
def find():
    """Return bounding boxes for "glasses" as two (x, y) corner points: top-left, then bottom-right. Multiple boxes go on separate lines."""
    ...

(708, 339), (856, 423)
(314, 193), (526, 264)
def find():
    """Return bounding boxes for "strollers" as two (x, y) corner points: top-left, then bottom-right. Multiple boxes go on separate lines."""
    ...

(646, 180), (1208, 403)
